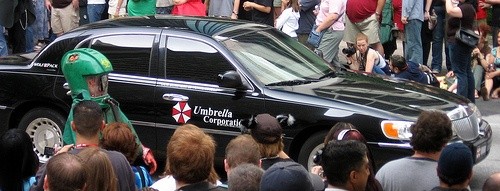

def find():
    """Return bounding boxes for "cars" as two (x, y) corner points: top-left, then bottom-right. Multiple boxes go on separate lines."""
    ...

(0, 13), (493, 174)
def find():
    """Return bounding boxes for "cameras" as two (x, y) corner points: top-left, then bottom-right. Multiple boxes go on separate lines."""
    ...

(342, 47), (355, 56)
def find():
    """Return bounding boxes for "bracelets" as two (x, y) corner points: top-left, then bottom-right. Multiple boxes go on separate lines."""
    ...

(117, 6), (121, 11)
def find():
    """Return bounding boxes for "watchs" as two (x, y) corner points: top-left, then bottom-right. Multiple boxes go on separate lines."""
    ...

(232, 10), (239, 16)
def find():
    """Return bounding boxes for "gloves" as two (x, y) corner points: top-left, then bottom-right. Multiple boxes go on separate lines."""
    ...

(141, 145), (157, 175)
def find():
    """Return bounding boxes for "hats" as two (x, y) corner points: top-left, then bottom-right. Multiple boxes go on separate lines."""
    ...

(251, 114), (281, 143)
(436, 143), (473, 186)
(259, 160), (325, 191)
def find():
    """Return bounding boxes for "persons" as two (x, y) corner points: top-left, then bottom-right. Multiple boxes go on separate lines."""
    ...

(1, 100), (500, 191)
(57, 48), (157, 175)
(1, 0), (500, 104)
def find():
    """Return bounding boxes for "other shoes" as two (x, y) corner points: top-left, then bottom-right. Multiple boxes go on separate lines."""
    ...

(433, 68), (440, 73)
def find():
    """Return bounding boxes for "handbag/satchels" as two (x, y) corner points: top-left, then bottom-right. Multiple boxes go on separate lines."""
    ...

(419, 63), (440, 87)
(455, 26), (479, 47)
(445, 76), (455, 86)
(306, 24), (325, 48)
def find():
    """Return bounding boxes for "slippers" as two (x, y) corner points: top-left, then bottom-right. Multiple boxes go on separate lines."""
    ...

(481, 86), (489, 101)
(491, 93), (498, 99)
